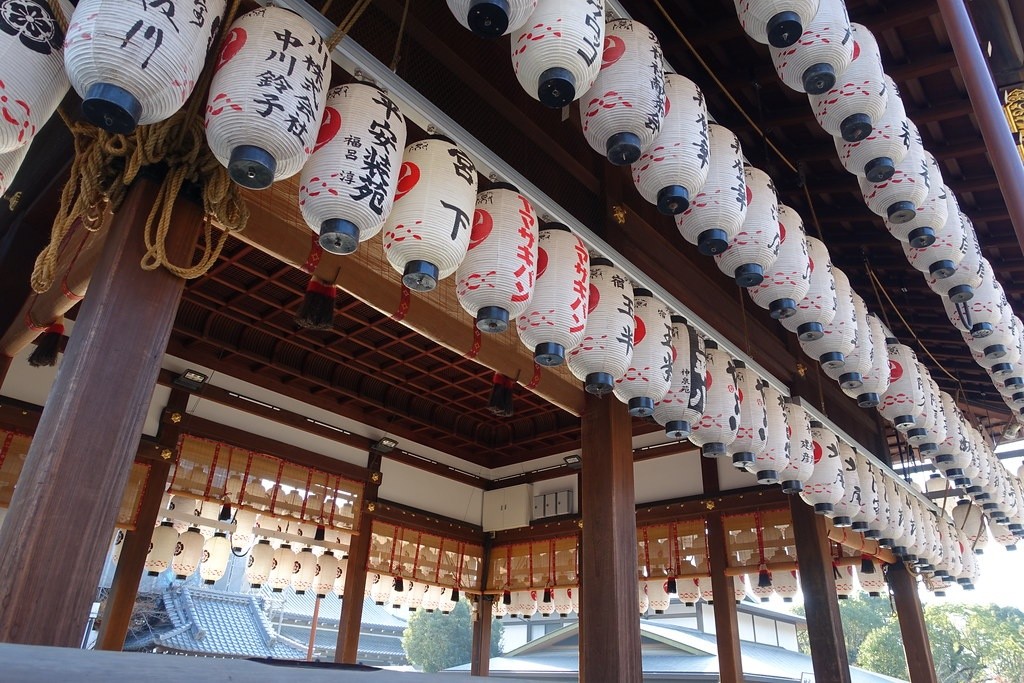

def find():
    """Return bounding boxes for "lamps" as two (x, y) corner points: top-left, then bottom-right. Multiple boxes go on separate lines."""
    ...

(563, 455), (584, 470)
(543, 583), (552, 603)
(451, 583), (461, 603)
(394, 574), (405, 593)
(173, 368), (209, 393)
(503, 587), (512, 605)
(313, 515), (325, 542)
(218, 494), (231, 523)
(667, 573), (677, 595)
(371, 437), (399, 454)
(1000, 421), (1022, 442)
(758, 564), (772, 588)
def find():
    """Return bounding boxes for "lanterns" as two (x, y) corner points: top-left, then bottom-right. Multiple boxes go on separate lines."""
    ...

(383, 134), (481, 295)
(111, 462), (649, 618)
(456, 179), (542, 335)
(204, 7), (334, 192)
(444, 0), (666, 165)
(0, 0), (76, 195)
(652, 0), (1024, 616)
(613, 288), (675, 417)
(60, 0), (228, 135)
(566, 259), (639, 396)
(299, 82), (410, 254)
(515, 223), (591, 368)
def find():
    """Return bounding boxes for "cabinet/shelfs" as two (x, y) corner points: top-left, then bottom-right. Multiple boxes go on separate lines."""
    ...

(482, 482), (534, 533)
